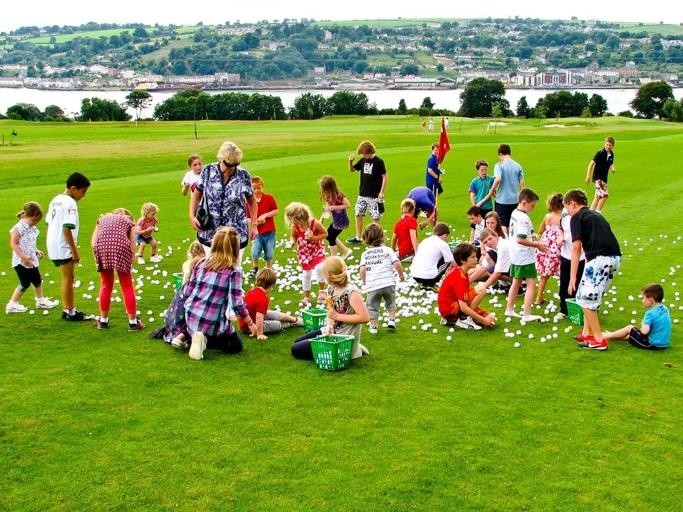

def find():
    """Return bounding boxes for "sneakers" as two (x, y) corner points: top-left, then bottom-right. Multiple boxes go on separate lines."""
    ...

(421, 284), (609, 351)
(138, 256), (145, 265)
(347, 238), (363, 243)
(400, 256), (414, 262)
(5, 297), (304, 360)
(317, 289), (328, 304)
(343, 249), (352, 261)
(369, 325), (378, 334)
(248, 267), (258, 279)
(388, 317), (395, 328)
(150, 254), (161, 262)
(299, 297), (311, 307)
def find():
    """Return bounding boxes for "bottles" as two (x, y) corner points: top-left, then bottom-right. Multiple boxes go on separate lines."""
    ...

(322, 205), (332, 219)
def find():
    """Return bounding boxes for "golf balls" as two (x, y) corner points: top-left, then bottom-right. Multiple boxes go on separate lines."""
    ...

(241, 220), (455, 340)
(454, 233), (469, 243)
(0, 237), (191, 324)
(603, 233), (683, 324)
(474, 274), (585, 348)
(316, 348), (351, 371)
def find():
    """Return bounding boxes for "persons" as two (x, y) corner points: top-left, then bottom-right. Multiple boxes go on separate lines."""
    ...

(134, 202), (162, 265)
(44, 171), (92, 322)
(5, 200), (59, 315)
(150, 117), (621, 360)
(90, 207), (146, 333)
(599, 284), (673, 350)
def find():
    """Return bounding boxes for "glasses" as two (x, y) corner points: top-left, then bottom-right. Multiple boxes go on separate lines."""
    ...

(223, 160), (240, 168)
